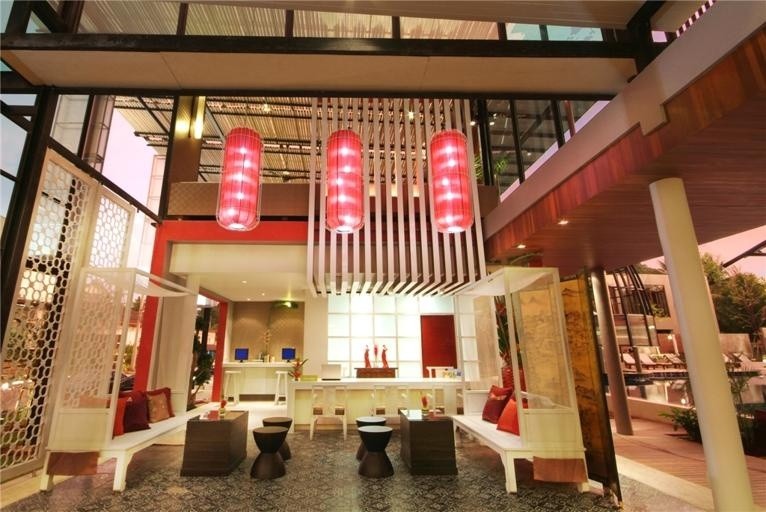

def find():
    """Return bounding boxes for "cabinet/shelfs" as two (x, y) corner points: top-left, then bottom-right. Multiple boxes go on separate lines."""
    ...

(354, 367), (397, 378)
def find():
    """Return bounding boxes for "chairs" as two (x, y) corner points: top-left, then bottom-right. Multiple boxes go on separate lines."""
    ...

(621, 348), (766, 371)
(368, 383), (410, 424)
(425, 366), (454, 379)
(308, 383), (350, 441)
(428, 383), (468, 448)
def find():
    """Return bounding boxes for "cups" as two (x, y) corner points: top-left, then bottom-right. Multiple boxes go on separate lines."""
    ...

(422, 406), (445, 417)
(202, 408), (225, 419)
(263, 354), (276, 362)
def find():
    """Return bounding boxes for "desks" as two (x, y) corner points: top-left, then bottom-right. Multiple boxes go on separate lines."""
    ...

(182, 408), (248, 476)
(285, 380), (476, 442)
(401, 409), (459, 475)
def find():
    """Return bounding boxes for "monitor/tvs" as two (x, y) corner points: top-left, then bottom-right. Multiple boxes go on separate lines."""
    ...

(282, 348), (295, 362)
(235, 348), (248, 362)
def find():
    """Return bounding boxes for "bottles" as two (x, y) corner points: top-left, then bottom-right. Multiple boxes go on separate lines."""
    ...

(443, 368), (462, 380)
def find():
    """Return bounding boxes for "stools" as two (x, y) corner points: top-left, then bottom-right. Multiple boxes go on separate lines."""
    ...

(357, 425), (394, 478)
(355, 416), (386, 459)
(224, 371), (242, 405)
(250, 426), (287, 480)
(274, 370), (290, 405)
(262, 416), (293, 459)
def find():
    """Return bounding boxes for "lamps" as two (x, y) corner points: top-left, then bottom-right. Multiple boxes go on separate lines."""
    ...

(234, 347), (249, 362)
(281, 346), (295, 363)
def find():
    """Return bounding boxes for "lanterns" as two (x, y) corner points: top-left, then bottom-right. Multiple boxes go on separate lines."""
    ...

(429, 129), (475, 235)
(215, 126), (264, 232)
(327, 129), (364, 234)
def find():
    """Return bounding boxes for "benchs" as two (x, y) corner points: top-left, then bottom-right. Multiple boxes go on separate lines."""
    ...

(42, 264), (200, 491)
(450, 264), (596, 496)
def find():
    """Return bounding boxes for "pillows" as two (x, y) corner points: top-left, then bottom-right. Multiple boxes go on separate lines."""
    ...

(107, 398), (126, 437)
(123, 400), (151, 433)
(118, 390), (143, 401)
(496, 398), (527, 435)
(143, 387), (174, 424)
(481, 384), (513, 424)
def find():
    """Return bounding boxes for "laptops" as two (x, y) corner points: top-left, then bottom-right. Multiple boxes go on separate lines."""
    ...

(321, 364), (341, 380)
